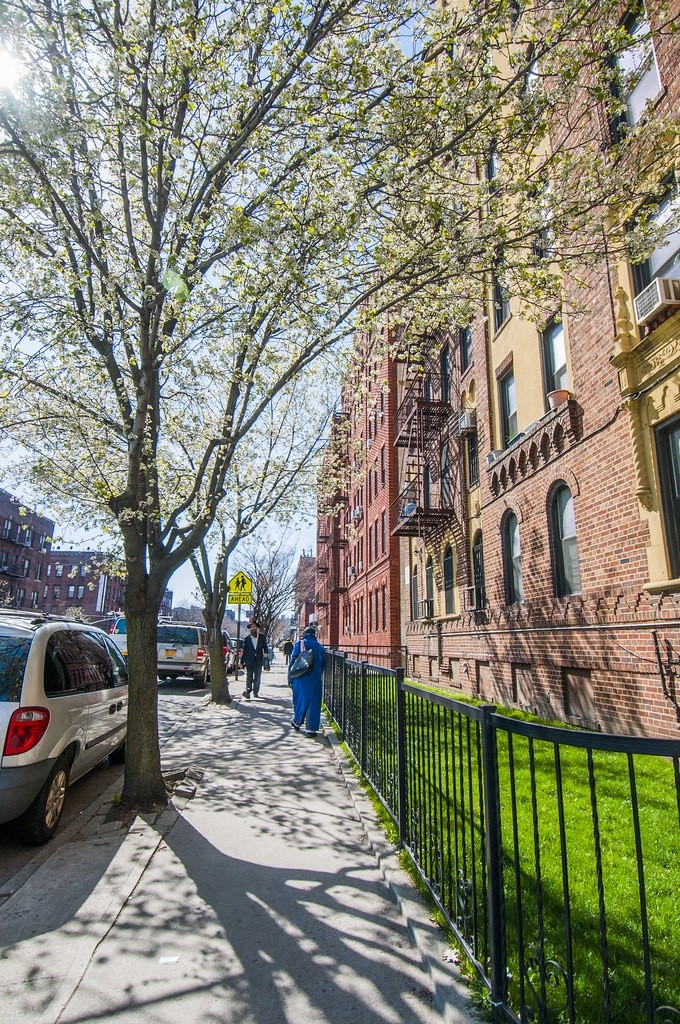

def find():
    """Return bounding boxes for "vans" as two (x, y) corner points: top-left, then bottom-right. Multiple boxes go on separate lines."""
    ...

(0, 608), (130, 847)
(108, 615), (162, 656)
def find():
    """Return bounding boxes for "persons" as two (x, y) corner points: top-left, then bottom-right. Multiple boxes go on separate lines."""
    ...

(240, 620), (269, 698)
(283, 638), (294, 665)
(288, 627), (326, 737)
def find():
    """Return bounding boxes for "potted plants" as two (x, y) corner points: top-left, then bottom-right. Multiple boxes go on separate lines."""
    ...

(506, 431), (525, 448)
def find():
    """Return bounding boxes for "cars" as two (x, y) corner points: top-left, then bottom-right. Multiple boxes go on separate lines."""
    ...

(222, 628), (245, 674)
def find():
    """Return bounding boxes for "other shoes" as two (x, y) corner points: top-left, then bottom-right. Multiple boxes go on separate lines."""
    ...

(253, 692), (258, 697)
(243, 691), (250, 699)
(290, 719), (300, 731)
(307, 732), (317, 738)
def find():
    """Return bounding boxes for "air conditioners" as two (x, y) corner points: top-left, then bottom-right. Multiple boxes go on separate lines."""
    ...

(356, 506), (363, 514)
(344, 626), (351, 634)
(350, 510), (360, 520)
(632, 277), (680, 327)
(457, 412), (476, 430)
(347, 567), (355, 576)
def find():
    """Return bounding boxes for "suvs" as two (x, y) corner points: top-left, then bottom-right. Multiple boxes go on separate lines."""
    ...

(157, 620), (211, 689)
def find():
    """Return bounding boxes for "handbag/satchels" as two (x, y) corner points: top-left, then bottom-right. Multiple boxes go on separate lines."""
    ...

(289, 640), (314, 678)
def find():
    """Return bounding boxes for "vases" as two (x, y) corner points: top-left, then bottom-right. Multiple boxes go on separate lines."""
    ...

(492, 450), (503, 459)
(546, 390), (569, 410)
(486, 455), (493, 464)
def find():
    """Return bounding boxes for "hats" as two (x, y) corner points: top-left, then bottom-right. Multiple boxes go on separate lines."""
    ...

(303, 627), (314, 636)
(247, 621), (260, 629)
